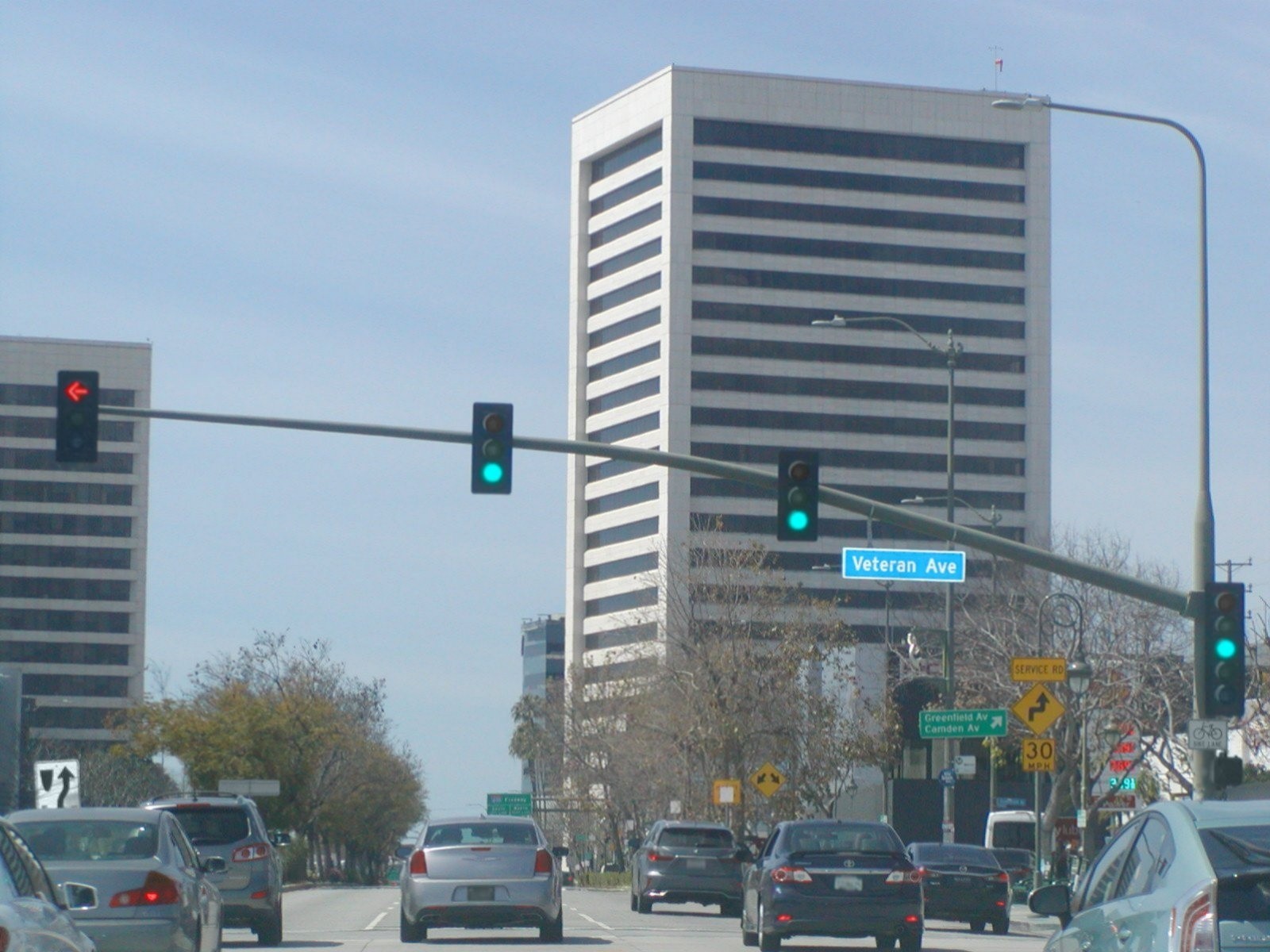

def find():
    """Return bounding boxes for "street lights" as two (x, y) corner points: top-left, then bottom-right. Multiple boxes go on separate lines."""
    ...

(900, 493), (1004, 597)
(990, 91), (1219, 797)
(1027, 798), (1270, 952)
(810, 312), (965, 847)
(1032, 591), (1093, 887)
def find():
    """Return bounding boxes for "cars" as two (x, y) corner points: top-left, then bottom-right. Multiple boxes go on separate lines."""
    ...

(3, 805), (227, 951)
(907, 842), (1013, 935)
(627, 819), (744, 918)
(992, 847), (1036, 889)
(394, 813), (570, 944)
(733, 819), (925, 952)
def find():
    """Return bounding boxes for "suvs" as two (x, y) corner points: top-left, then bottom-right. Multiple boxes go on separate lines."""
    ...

(137, 789), (291, 946)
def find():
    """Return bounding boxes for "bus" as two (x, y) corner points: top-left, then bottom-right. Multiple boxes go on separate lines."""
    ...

(984, 809), (1045, 852)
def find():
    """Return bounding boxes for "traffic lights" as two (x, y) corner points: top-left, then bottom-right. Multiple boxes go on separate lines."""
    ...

(53, 369), (101, 464)
(470, 402), (514, 495)
(776, 447), (821, 542)
(1203, 581), (1247, 718)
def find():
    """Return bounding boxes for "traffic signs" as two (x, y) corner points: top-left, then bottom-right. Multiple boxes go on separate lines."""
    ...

(918, 707), (1009, 740)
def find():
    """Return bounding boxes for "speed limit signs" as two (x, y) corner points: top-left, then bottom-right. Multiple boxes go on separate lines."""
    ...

(1021, 738), (1056, 772)
